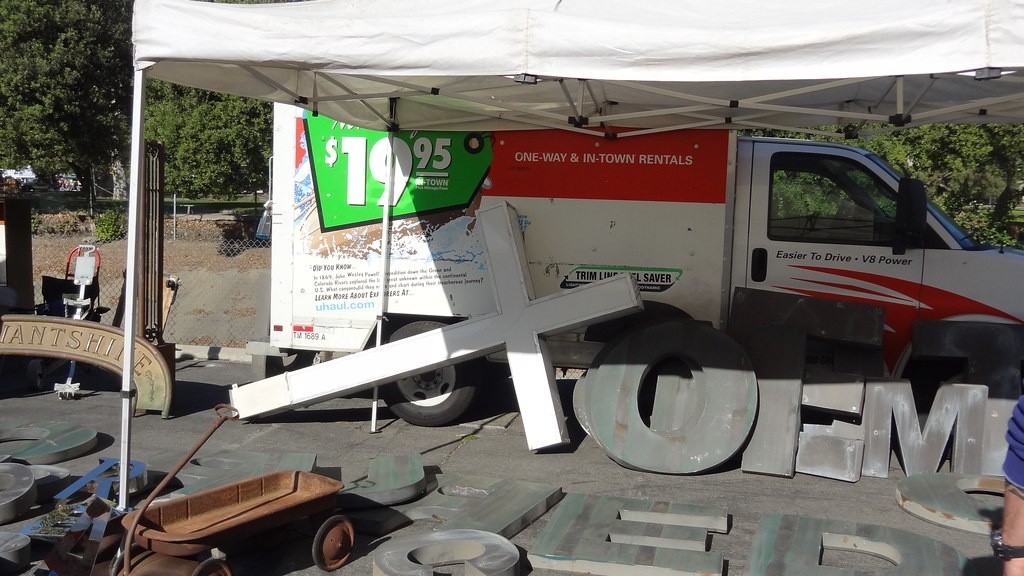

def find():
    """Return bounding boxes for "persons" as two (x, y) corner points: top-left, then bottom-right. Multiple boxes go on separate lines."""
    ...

(989, 393), (1024, 576)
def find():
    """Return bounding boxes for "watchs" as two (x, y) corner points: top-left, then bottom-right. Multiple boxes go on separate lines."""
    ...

(991, 527), (1024, 561)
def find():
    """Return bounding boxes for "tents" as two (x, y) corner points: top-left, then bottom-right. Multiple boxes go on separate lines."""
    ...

(114, 1), (1024, 513)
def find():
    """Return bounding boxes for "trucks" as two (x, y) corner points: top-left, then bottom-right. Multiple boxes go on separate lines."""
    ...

(257, 99), (1024, 426)
